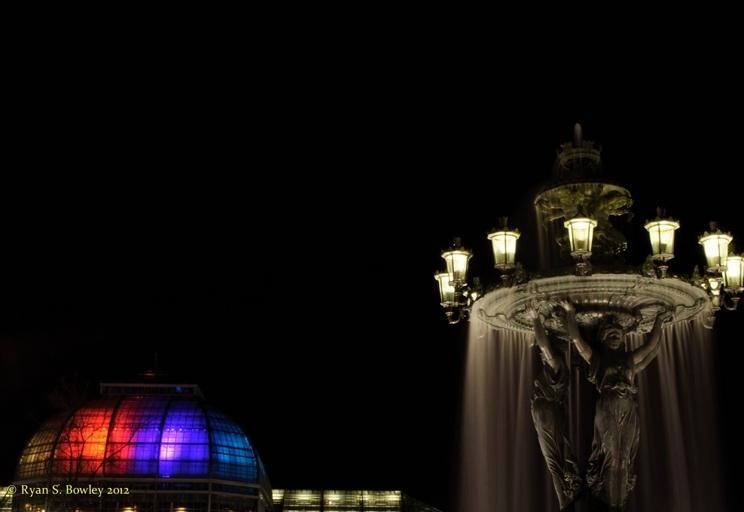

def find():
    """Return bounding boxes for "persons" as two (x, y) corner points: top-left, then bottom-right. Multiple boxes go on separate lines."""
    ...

(625, 316), (674, 492)
(550, 296), (674, 512)
(520, 302), (592, 512)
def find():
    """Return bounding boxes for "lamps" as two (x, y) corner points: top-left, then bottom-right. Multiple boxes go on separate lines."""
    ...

(429, 202), (743, 327)
